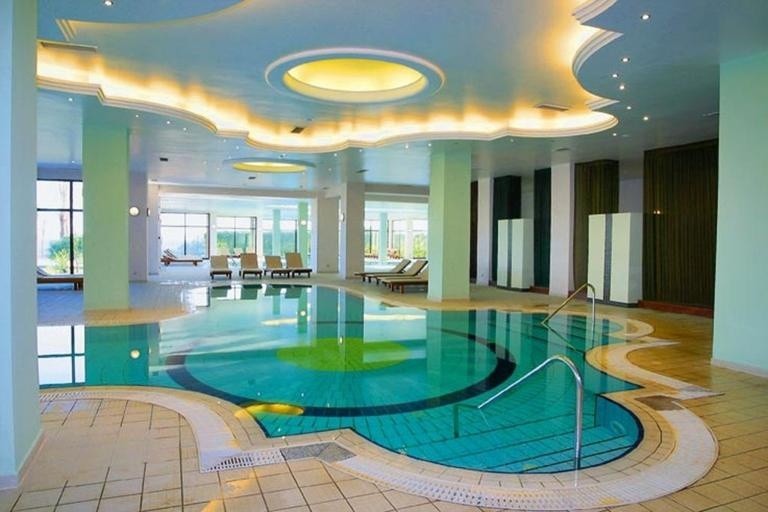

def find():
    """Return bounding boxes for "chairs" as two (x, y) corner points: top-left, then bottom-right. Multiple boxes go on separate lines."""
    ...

(354, 257), (429, 294)
(209, 252), (312, 279)
(160, 249), (202, 266)
(35, 266), (83, 290)
